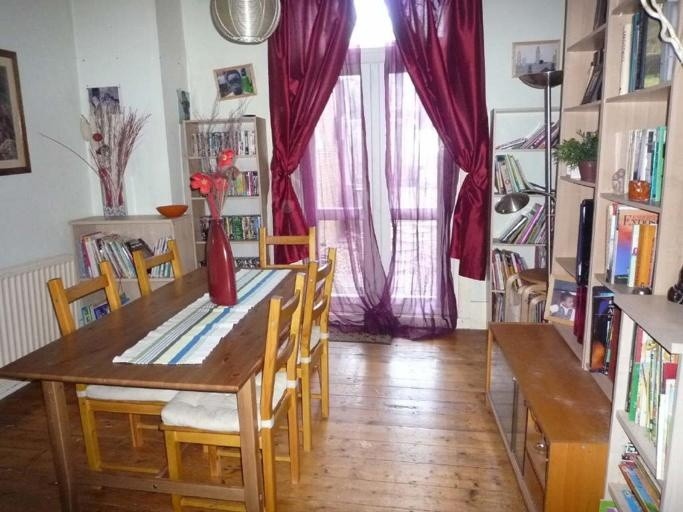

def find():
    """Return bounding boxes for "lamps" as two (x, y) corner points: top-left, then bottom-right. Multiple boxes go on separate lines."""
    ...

(518, 69), (563, 288)
(210, 1), (281, 45)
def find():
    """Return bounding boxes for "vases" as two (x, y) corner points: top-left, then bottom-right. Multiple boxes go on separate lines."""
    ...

(205, 220), (243, 308)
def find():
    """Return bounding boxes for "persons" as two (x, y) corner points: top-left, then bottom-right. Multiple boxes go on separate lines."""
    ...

(220, 70), (251, 98)
(549, 291), (574, 321)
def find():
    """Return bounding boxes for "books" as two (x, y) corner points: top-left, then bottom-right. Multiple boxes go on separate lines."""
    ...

(190, 125), (262, 270)
(574, 0), (678, 382)
(492, 111), (559, 322)
(594, 322), (678, 512)
(79, 230), (177, 328)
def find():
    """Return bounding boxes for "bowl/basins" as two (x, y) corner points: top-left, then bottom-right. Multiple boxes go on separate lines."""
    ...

(156, 205), (188, 218)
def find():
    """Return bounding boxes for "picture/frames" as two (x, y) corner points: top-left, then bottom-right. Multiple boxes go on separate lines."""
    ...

(213, 63), (258, 102)
(0, 48), (32, 180)
(511, 39), (561, 79)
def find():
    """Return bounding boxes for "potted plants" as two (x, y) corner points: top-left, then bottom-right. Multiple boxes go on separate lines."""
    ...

(37, 108), (153, 218)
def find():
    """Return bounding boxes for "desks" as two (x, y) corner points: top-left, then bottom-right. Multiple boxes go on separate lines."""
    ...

(0, 259), (319, 511)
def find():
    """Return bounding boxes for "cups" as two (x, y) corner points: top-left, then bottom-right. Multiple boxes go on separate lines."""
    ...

(628, 180), (649, 201)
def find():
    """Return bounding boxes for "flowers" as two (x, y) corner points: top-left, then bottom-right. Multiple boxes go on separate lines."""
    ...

(188, 102), (247, 218)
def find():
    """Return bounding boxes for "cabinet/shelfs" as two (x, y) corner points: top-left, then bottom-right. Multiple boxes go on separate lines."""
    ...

(541, 2), (683, 406)
(484, 107), (559, 321)
(183, 117), (269, 267)
(599, 295), (683, 512)
(68, 215), (199, 325)
(483, 321), (613, 512)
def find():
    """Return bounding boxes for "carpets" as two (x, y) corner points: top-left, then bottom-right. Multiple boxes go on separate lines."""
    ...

(328, 324), (392, 345)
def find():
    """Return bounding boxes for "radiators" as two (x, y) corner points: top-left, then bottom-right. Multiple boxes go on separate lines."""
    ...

(1, 252), (79, 399)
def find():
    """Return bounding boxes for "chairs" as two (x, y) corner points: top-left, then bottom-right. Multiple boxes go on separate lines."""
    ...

(48, 263), (165, 492)
(157, 268), (306, 511)
(297, 248), (336, 453)
(259, 226), (317, 271)
